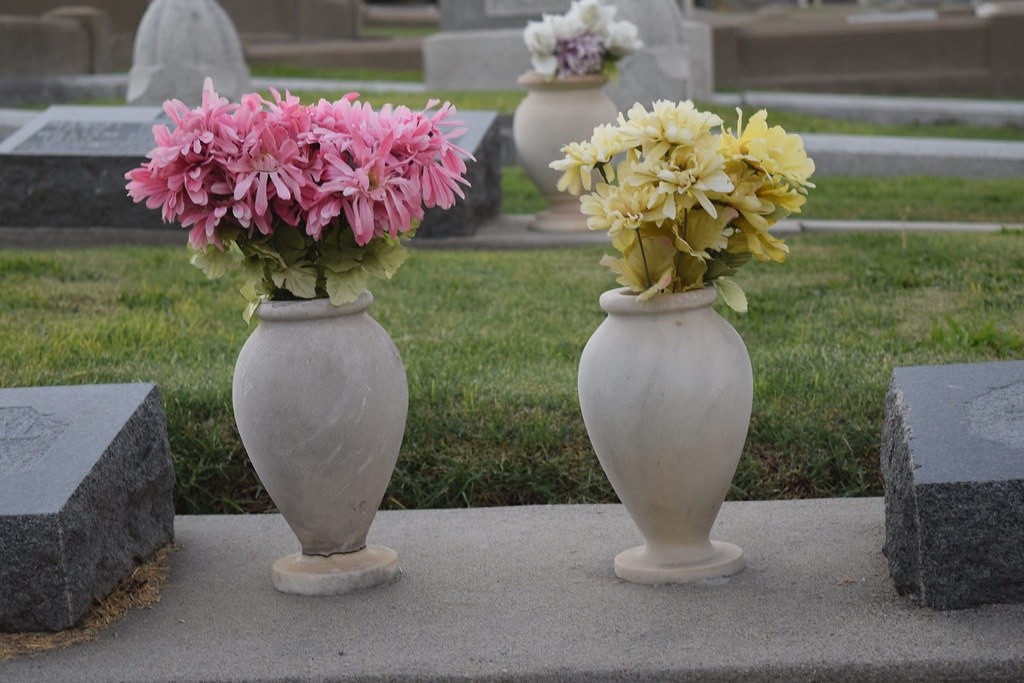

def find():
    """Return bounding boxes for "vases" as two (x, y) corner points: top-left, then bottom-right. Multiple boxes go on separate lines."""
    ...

(512, 72), (624, 232)
(577, 283), (754, 585)
(231, 290), (409, 596)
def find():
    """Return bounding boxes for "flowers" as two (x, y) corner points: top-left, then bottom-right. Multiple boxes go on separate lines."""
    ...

(524, 0), (644, 83)
(549, 97), (817, 313)
(122, 77), (478, 323)
